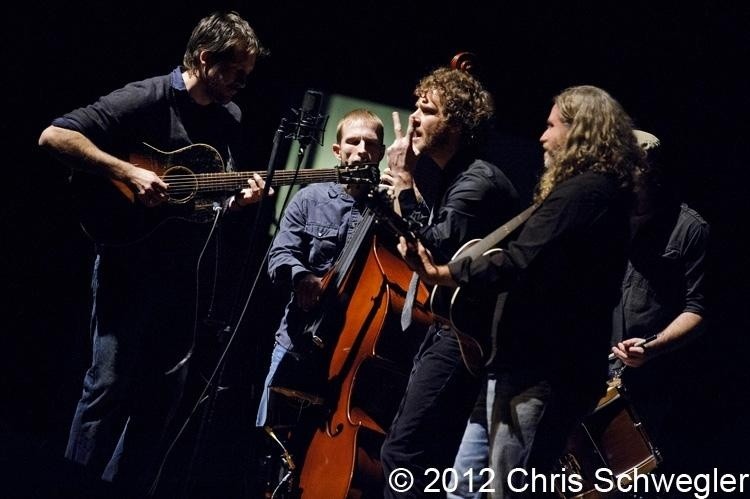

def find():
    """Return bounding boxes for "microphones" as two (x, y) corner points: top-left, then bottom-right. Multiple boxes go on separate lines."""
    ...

(293, 87), (326, 150)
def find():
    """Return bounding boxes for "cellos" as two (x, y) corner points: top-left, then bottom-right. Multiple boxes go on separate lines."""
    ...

(267, 47), (474, 498)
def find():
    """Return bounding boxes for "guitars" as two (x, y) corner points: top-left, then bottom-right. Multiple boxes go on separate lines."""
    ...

(65, 126), (379, 258)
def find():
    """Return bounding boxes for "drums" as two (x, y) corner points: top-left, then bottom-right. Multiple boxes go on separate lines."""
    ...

(550, 386), (662, 499)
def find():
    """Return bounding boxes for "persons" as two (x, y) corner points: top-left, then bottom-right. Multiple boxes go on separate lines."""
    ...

(395, 83), (647, 499)
(584, 129), (722, 498)
(36, 11), (274, 498)
(254, 107), (391, 498)
(371, 65), (521, 499)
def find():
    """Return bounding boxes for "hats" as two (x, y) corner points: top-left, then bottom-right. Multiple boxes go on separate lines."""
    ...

(634, 130), (662, 155)
